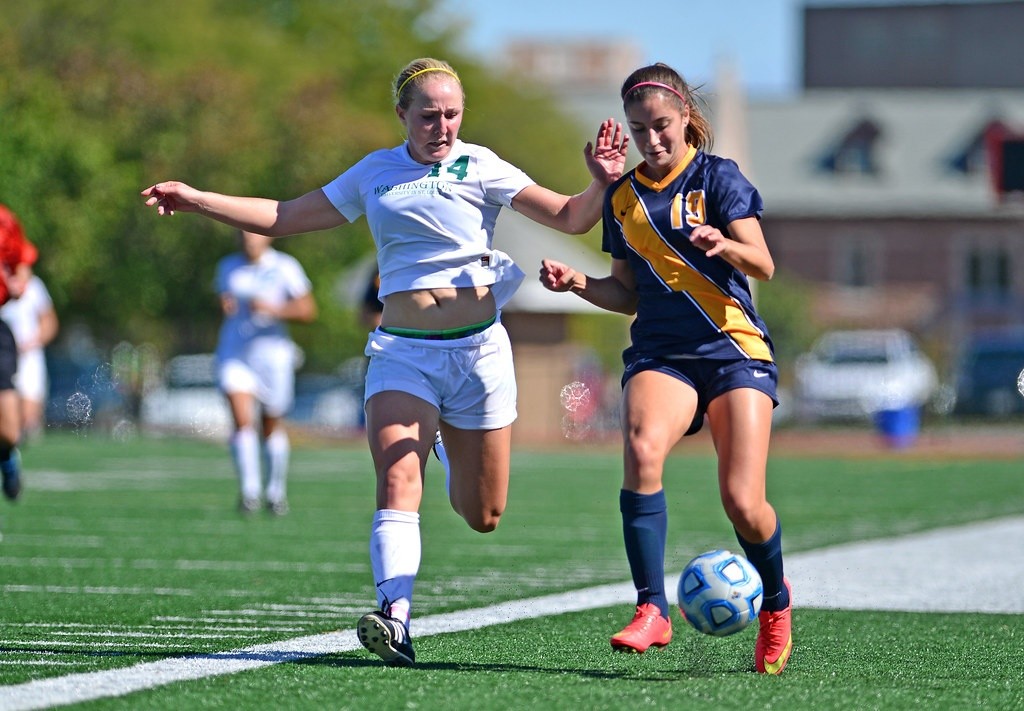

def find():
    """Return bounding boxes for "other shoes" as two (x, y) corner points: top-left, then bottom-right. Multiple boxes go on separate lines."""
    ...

(238, 500), (290, 515)
(0, 448), (22, 499)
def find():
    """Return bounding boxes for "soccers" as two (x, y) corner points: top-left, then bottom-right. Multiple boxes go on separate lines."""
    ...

(677, 549), (764, 637)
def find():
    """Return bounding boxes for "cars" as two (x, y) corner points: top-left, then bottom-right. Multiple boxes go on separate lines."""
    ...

(48, 353), (125, 432)
(793, 328), (940, 428)
(954, 326), (1024, 426)
(140, 356), (232, 448)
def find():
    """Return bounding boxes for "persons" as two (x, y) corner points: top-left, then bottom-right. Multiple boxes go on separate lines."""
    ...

(0, 205), (58, 503)
(538, 64), (796, 676)
(141, 56), (632, 668)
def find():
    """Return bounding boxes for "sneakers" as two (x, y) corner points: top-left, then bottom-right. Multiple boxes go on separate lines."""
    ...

(610, 603), (672, 653)
(357, 611), (415, 668)
(755, 578), (792, 675)
(432, 430), (444, 462)
